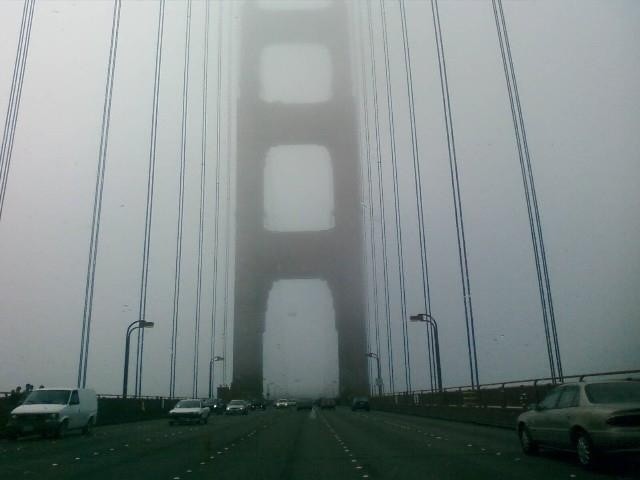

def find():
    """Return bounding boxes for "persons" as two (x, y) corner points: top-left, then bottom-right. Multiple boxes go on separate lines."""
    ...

(7, 383), (45, 399)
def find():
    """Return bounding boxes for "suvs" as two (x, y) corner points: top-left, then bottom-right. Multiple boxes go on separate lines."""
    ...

(202, 398), (225, 415)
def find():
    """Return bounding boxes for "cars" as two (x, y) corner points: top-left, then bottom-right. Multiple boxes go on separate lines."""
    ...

(225, 399), (247, 416)
(275, 395), (337, 412)
(250, 400), (265, 410)
(517, 379), (640, 468)
(169, 399), (210, 426)
(351, 395), (370, 411)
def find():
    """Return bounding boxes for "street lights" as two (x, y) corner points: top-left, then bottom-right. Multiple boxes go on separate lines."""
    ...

(365, 351), (382, 396)
(209, 355), (224, 399)
(123, 318), (155, 400)
(411, 312), (441, 392)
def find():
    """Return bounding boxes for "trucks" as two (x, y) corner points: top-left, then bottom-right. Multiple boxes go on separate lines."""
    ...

(8, 388), (99, 438)
